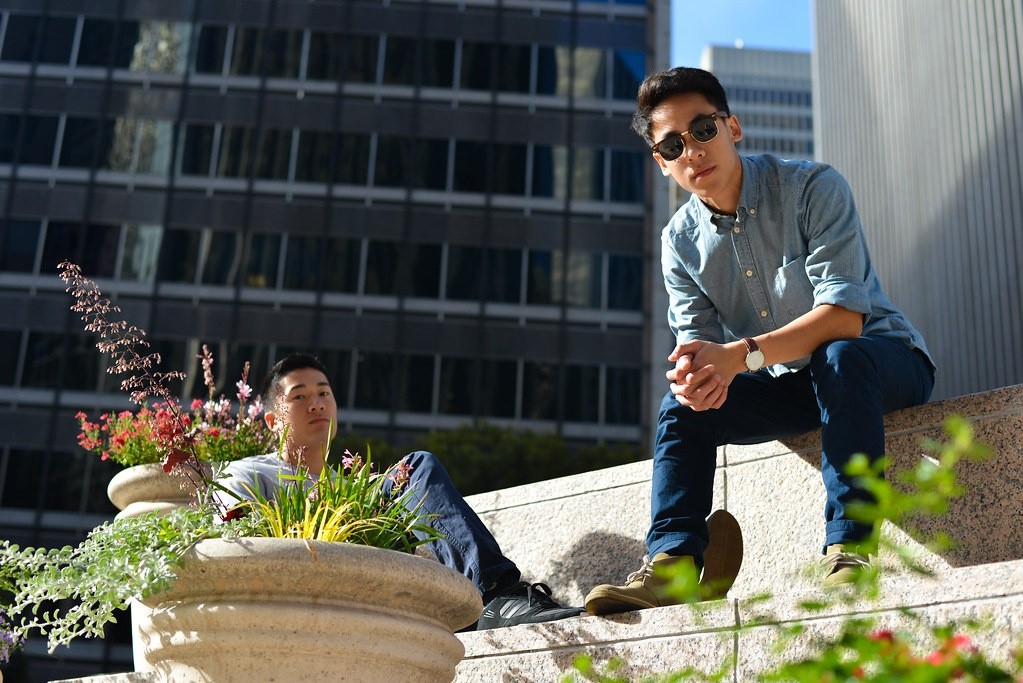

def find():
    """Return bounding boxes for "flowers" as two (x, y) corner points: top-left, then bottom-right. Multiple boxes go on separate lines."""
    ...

(76, 396), (271, 478)
(1, 452), (485, 660)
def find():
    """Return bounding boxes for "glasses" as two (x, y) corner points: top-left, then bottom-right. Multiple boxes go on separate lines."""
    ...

(649, 109), (734, 162)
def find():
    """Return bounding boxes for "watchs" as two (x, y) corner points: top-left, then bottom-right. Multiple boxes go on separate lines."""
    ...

(741, 337), (765, 373)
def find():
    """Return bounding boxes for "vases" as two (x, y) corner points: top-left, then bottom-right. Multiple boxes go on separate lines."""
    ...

(126, 538), (485, 681)
(106, 457), (211, 532)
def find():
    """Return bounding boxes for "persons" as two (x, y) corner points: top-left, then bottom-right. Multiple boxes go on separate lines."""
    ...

(213, 354), (588, 630)
(584, 66), (938, 617)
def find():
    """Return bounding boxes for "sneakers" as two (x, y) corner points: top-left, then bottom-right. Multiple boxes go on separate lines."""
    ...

(475, 580), (589, 631)
(584, 549), (708, 614)
(698, 509), (744, 604)
(817, 544), (875, 587)
